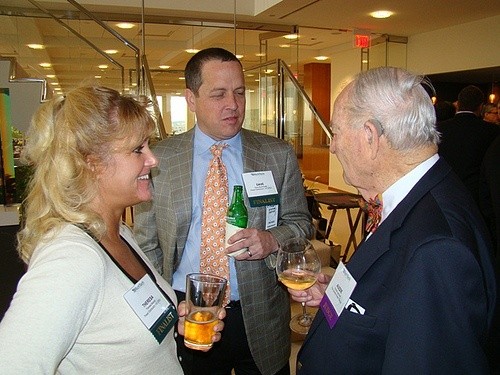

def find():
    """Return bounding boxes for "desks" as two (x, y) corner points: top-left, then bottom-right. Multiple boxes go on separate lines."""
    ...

(315, 193), (370, 264)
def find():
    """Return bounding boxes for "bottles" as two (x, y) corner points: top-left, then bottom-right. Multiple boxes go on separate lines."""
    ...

(225, 185), (248, 257)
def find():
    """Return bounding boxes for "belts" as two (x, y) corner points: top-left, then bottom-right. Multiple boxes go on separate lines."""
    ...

(173, 289), (243, 311)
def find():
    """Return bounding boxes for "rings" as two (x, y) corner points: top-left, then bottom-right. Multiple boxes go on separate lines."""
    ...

(246, 249), (252, 257)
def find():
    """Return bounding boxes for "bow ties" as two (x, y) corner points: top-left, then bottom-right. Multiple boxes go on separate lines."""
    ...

(357, 192), (383, 234)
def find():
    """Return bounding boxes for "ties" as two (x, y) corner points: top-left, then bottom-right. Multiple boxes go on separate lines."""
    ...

(199, 144), (230, 309)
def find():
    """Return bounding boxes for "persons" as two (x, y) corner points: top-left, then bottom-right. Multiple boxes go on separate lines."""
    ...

(436, 101), (456, 120)
(437, 85), (500, 172)
(478, 135), (500, 235)
(1, 81), (226, 375)
(296, 68), (500, 375)
(132, 48), (313, 375)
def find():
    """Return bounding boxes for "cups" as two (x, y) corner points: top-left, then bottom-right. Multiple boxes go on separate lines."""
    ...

(183, 272), (227, 351)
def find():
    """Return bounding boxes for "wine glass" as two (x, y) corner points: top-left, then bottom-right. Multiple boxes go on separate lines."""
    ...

(275, 238), (321, 335)
